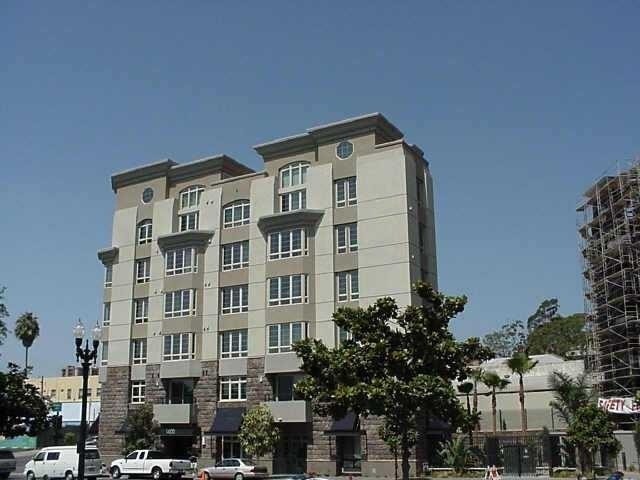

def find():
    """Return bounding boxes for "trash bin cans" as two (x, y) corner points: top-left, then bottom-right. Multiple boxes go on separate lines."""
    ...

(423, 462), (428, 473)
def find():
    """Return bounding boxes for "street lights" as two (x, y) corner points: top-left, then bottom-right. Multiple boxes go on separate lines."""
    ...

(71, 317), (103, 480)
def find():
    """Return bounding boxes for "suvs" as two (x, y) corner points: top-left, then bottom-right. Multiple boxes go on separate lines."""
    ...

(24, 445), (103, 479)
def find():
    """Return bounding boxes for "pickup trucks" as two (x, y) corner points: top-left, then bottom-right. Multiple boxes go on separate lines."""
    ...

(108, 450), (192, 479)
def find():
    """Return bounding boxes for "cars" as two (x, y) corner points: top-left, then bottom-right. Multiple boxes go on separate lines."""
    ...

(0, 449), (17, 479)
(75, 436), (98, 445)
(198, 457), (268, 480)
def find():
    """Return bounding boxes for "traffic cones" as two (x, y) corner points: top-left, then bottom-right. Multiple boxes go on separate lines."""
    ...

(201, 472), (206, 480)
